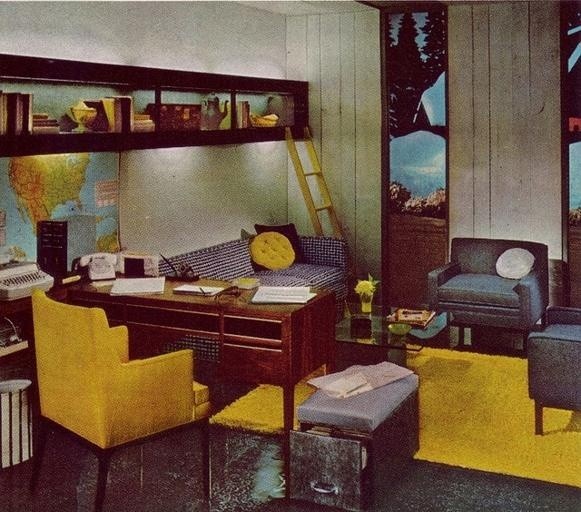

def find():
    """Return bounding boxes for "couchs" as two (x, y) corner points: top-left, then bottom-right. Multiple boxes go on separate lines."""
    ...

(158, 234), (347, 322)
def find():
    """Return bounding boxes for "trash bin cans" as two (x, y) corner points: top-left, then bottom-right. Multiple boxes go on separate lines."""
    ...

(0, 379), (34, 468)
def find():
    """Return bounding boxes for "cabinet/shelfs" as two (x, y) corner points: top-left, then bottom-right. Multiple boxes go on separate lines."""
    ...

(1, 53), (311, 158)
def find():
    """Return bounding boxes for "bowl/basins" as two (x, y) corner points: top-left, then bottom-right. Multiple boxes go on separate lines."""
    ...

(388, 323), (412, 336)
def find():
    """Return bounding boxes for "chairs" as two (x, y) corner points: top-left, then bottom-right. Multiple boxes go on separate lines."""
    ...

(527, 304), (580, 435)
(27, 287), (211, 511)
(428, 237), (549, 342)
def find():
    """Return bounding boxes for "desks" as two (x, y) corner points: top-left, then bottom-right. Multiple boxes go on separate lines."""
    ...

(67, 275), (335, 501)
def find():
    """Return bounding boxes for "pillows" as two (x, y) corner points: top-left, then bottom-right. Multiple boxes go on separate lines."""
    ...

(254, 223), (303, 264)
(248, 231), (295, 270)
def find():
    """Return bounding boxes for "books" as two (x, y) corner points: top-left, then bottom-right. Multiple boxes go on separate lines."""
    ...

(250, 285), (317, 304)
(109, 275), (166, 298)
(230, 277), (260, 289)
(237, 99), (252, 130)
(1, 85), (137, 147)
(172, 283), (226, 297)
(384, 306), (437, 329)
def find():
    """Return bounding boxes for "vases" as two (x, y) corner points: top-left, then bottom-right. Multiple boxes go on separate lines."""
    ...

(361, 301), (373, 311)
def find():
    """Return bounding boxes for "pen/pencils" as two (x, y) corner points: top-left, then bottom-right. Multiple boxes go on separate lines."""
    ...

(347, 383), (367, 393)
(159, 253), (176, 273)
(200, 287), (205, 294)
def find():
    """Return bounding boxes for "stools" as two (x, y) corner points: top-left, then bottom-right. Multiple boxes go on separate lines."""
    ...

(298, 366), (419, 510)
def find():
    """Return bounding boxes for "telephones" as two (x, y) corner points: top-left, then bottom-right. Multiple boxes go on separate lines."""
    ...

(79, 252), (117, 280)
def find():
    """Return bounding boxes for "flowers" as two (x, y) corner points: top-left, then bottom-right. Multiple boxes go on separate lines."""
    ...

(355, 274), (381, 301)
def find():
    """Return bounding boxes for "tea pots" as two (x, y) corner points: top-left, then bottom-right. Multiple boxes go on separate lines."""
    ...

(199, 90), (229, 131)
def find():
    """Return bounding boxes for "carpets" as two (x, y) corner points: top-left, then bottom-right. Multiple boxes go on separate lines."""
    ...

(209, 344), (581, 489)
